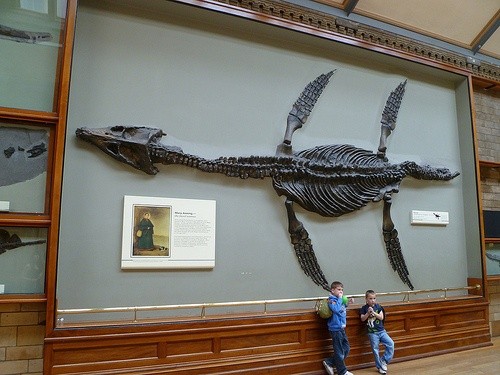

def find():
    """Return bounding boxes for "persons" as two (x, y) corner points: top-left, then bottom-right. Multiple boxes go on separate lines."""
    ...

(323, 281), (354, 375)
(137, 213), (154, 251)
(360, 290), (394, 374)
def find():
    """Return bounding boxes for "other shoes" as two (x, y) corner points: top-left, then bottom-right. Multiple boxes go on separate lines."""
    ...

(379, 359), (387, 371)
(344, 371), (355, 375)
(377, 367), (386, 374)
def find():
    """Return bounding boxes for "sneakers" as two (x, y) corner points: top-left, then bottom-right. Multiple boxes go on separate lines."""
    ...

(323, 360), (335, 375)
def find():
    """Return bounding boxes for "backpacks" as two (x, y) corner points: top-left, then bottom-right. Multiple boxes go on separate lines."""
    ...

(315, 298), (333, 319)
(378, 305), (385, 326)
